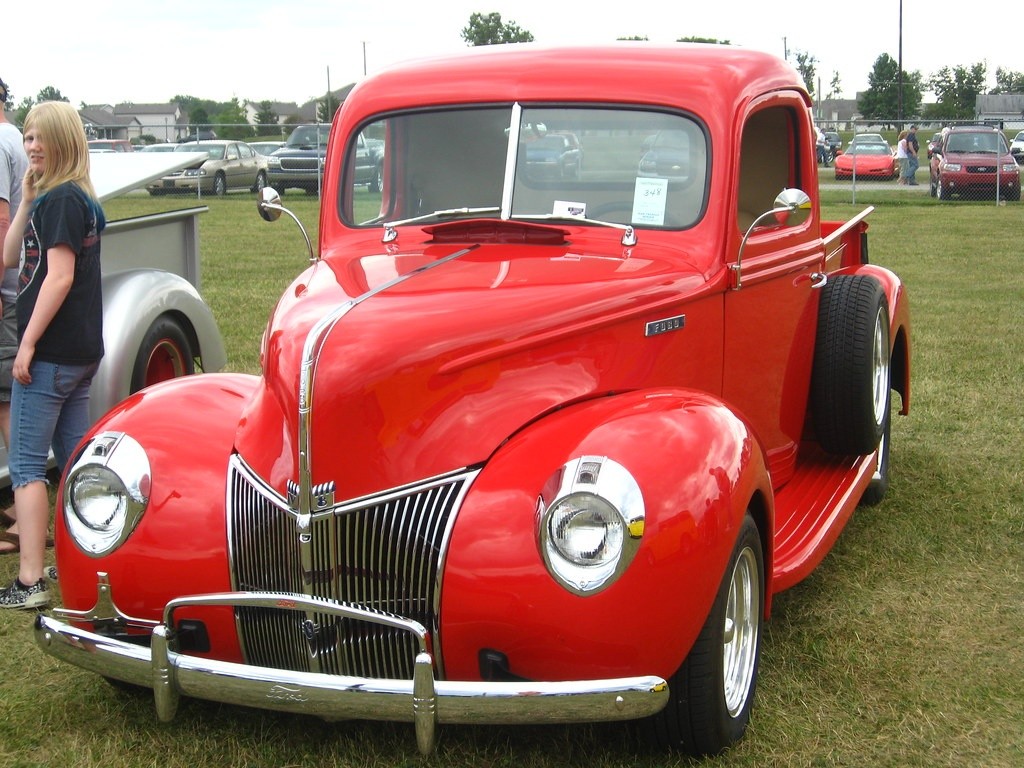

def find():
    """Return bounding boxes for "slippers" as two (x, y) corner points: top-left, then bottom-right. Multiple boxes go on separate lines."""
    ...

(0, 530), (54, 555)
(0, 506), (52, 532)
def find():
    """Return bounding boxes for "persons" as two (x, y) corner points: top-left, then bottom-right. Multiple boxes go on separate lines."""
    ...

(816, 128), (830, 167)
(896, 125), (920, 185)
(0, 75), (105, 608)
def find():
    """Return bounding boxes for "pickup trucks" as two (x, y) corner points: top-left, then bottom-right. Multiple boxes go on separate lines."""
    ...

(0, 204), (224, 497)
(264, 121), (385, 197)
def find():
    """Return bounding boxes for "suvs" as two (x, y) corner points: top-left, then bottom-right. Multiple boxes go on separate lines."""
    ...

(928, 125), (1021, 202)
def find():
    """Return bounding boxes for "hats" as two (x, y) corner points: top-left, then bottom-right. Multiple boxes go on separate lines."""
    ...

(910, 126), (918, 129)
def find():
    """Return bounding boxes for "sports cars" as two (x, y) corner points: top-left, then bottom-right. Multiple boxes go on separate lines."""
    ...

(833, 141), (897, 180)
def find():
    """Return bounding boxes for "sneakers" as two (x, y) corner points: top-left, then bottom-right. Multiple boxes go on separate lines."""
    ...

(43, 566), (57, 582)
(0, 577), (51, 609)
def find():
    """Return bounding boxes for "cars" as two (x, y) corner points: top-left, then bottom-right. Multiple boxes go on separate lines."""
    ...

(1009, 129), (1024, 162)
(87, 138), (134, 153)
(147, 138), (267, 197)
(824, 132), (843, 153)
(637, 127), (690, 187)
(245, 142), (288, 158)
(33, 40), (911, 755)
(522, 129), (583, 181)
(925, 133), (942, 159)
(187, 130), (216, 143)
(847, 133), (889, 151)
(138, 143), (180, 154)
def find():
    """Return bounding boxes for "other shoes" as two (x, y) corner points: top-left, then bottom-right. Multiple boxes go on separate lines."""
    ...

(825, 164), (831, 167)
(909, 181), (919, 185)
(897, 179), (905, 185)
